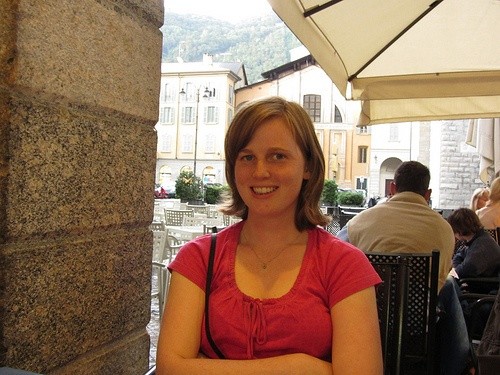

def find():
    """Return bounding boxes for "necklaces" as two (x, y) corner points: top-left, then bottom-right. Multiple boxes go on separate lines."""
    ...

(251, 235), (300, 269)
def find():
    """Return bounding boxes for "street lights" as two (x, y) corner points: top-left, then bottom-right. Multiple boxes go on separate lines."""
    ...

(178, 82), (211, 177)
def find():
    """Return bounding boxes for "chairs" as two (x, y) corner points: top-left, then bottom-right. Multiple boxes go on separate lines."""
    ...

(149, 202), (500, 375)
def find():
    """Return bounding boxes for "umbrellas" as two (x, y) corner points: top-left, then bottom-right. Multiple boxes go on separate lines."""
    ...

(465, 118), (500, 183)
(265, 0), (500, 127)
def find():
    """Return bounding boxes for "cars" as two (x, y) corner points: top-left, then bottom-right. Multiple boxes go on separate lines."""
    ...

(153, 180), (178, 199)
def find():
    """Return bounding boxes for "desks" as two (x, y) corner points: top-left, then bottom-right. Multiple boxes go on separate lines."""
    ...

(167, 226), (213, 240)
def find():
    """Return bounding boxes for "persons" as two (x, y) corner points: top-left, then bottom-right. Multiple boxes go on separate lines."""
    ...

(338, 160), (500, 375)
(161, 187), (165, 195)
(155, 95), (384, 374)
